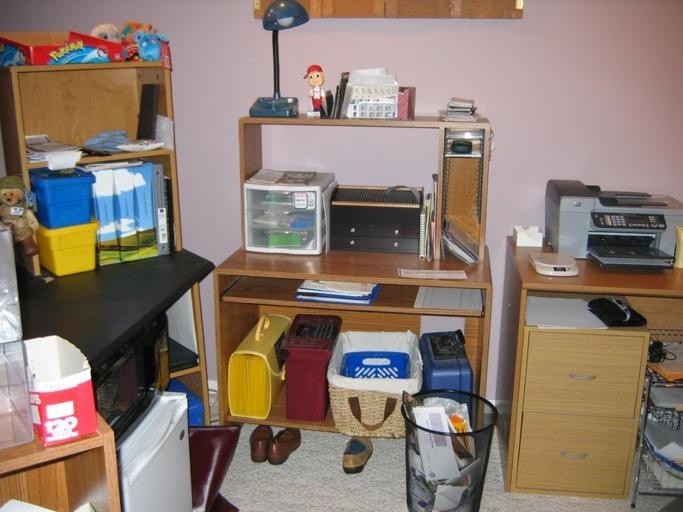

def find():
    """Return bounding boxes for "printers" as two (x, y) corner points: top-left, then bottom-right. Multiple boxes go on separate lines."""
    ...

(544, 179), (683, 270)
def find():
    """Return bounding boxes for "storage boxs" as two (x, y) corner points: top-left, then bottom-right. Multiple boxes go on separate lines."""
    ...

(38, 220), (100, 277)
(31, 168), (97, 226)
(417, 329), (473, 402)
(239, 166), (334, 257)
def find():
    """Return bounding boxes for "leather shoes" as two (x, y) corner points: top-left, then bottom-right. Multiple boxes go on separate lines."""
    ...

(268, 427), (301, 465)
(250, 425), (273, 462)
(342, 436), (373, 474)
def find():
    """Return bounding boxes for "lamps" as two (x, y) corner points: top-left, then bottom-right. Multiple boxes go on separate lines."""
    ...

(246, 2), (312, 117)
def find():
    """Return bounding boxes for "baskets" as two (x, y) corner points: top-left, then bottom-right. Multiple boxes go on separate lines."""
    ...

(327, 330), (423, 439)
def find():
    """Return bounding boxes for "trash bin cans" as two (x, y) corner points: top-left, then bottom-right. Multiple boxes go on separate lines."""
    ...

(401, 388), (498, 512)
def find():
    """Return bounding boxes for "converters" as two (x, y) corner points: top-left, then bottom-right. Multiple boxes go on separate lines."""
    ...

(649, 347), (663, 363)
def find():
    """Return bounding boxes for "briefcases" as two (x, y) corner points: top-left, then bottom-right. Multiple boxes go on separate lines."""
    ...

(228, 313), (291, 420)
(418, 330), (474, 422)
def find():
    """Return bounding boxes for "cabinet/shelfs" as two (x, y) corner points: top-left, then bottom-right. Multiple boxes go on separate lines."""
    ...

(200, 107), (494, 448)
(324, 183), (423, 260)
(0, 398), (124, 512)
(1, 41), (211, 430)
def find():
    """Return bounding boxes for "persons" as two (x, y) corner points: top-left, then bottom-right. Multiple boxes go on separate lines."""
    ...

(304, 64), (326, 112)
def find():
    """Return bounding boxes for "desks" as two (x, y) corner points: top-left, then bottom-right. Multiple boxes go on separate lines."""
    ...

(496, 230), (680, 501)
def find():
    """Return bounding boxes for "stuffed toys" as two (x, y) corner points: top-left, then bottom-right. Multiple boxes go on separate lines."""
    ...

(0, 175), (40, 256)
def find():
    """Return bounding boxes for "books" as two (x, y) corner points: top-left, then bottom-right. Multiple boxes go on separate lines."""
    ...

(443, 96), (479, 123)
(420, 203), (427, 257)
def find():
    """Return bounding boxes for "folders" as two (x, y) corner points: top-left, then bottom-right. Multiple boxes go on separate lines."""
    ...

(152, 163), (171, 256)
